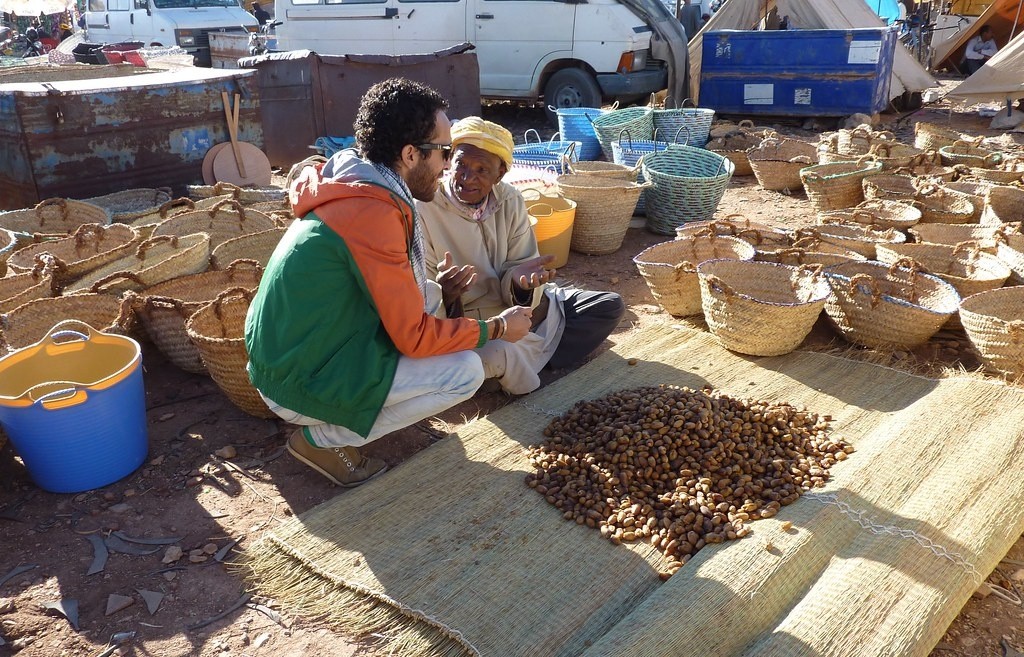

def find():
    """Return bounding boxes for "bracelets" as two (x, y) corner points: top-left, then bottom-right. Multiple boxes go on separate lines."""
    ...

(488, 317), (500, 340)
(498, 316), (508, 340)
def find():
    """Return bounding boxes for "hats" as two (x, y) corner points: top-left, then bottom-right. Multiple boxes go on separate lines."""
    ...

(449, 116), (514, 173)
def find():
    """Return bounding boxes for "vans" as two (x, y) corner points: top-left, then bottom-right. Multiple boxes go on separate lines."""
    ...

(274, 0), (686, 133)
(85, 0), (260, 68)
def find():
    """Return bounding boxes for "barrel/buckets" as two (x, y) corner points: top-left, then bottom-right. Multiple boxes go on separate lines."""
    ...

(0, 101), (1024, 495)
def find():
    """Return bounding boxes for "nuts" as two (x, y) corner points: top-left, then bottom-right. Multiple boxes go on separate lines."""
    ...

(524, 384), (856, 578)
(628, 360), (636, 365)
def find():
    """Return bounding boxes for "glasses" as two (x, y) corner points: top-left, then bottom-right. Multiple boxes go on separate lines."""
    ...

(413, 142), (453, 161)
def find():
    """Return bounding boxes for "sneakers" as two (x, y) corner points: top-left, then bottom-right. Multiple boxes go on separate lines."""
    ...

(285, 426), (388, 487)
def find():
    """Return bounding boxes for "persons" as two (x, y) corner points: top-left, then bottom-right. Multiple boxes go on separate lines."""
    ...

(702, 13), (710, 21)
(0, 23), (73, 55)
(964, 25), (999, 75)
(252, 2), (271, 25)
(679, 0), (701, 41)
(243, 76), (532, 487)
(413, 116), (624, 396)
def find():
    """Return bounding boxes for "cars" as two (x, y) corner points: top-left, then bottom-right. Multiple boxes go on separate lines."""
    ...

(0, 26), (14, 54)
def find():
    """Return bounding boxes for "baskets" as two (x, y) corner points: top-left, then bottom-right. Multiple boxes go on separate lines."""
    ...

(0, 93), (1024, 494)
